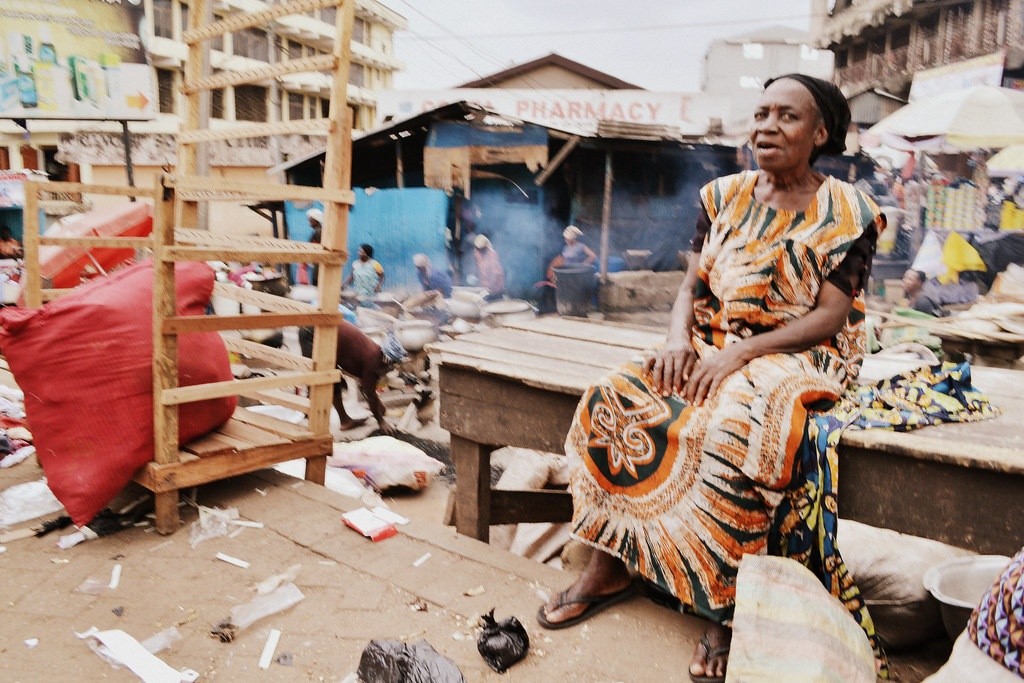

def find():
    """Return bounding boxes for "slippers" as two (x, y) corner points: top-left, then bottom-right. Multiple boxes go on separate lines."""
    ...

(536, 574), (637, 629)
(688, 627), (731, 682)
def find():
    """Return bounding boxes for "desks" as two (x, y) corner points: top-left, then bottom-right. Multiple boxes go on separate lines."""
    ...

(422, 313), (1024, 557)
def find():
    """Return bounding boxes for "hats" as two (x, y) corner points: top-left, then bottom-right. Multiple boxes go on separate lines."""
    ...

(382, 333), (408, 362)
(361, 244), (372, 256)
(305, 208), (322, 224)
(764, 73), (851, 155)
(563, 225), (583, 241)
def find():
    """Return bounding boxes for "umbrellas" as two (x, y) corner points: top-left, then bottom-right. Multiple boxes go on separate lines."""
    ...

(869, 81), (1024, 156)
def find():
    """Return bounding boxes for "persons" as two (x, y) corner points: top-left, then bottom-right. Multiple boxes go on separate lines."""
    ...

(0, 227), (23, 258)
(562, 225), (595, 266)
(536, 75), (888, 683)
(306, 208), (323, 285)
(474, 235), (504, 294)
(342, 245), (385, 293)
(414, 254), (451, 299)
(902, 269), (938, 315)
